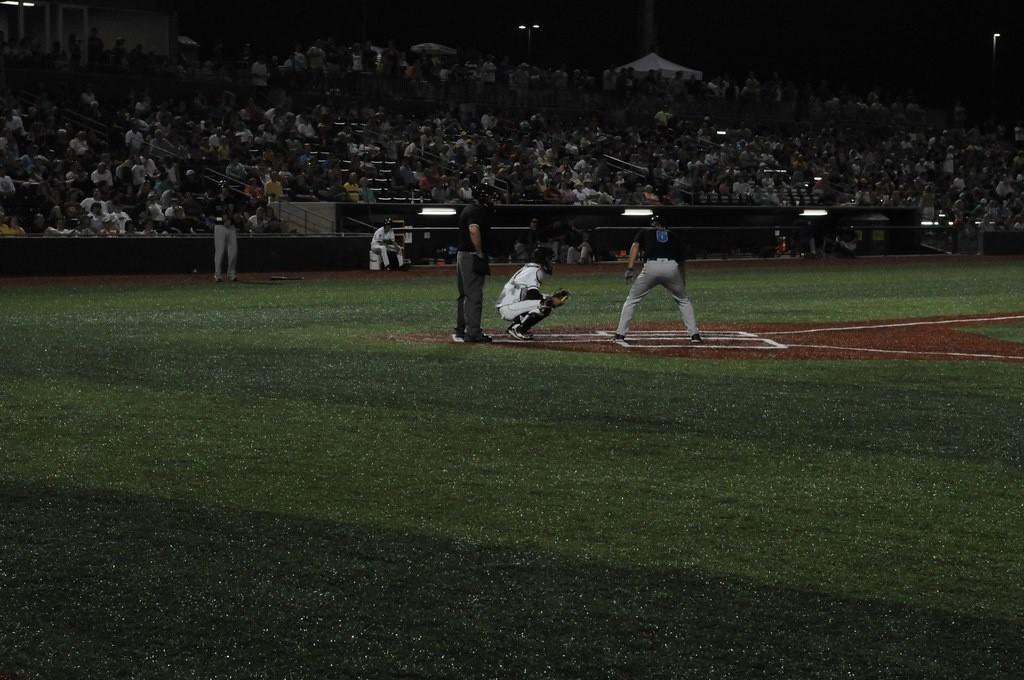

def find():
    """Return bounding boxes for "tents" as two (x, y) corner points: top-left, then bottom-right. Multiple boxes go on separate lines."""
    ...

(613, 51), (703, 82)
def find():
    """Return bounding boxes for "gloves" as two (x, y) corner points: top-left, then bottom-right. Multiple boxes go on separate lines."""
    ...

(624, 268), (634, 282)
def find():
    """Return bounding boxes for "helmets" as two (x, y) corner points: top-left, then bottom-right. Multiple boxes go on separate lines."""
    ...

(530, 246), (556, 274)
(649, 215), (664, 227)
(471, 182), (501, 216)
(383, 217), (392, 225)
(217, 179), (228, 189)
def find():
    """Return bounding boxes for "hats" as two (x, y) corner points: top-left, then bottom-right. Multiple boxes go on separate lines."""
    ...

(644, 184), (653, 189)
(486, 130), (493, 137)
(185, 169), (195, 175)
(461, 131), (467, 136)
(79, 214), (94, 224)
(514, 161), (520, 167)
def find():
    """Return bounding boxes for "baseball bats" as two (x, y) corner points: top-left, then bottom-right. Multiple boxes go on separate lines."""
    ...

(269, 276), (305, 280)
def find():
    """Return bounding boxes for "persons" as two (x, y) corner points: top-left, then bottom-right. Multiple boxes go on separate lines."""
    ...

(603, 65), (927, 205)
(344, 171), (362, 201)
(90, 162), (114, 187)
(280, 35), (611, 207)
(798, 222), (859, 259)
(495, 248), (565, 341)
(452, 183), (498, 341)
(149, 130), (175, 154)
(264, 170), (283, 201)
(612, 215), (704, 343)
(510, 62), (530, 109)
(124, 119), (145, 150)
(205, 180), (245, 282)
(913, 118), (1024, 231)
(480, 53), (497, 105)
(399, 155), (417, 185)
(0, 26), (290, 236)
(371, 217), (407, 271)
(514, 217), (598, 264)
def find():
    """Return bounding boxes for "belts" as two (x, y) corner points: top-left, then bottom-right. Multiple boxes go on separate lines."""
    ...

(647, 257), (675, 261)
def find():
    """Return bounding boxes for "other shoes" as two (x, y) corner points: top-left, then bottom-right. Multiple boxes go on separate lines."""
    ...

(456, 334), (465, 338)
(228, 275), (237, 281)
(614, 332), (625, 342)
(508, 323), (532, 340)
(385, 266), (393, 271)
(577, 258), (584, 264)
(399, 266), (407, 271)
(215, 276), (223, 282)
(464, 336), (493, 342)
(691, 334), (702, 343)
